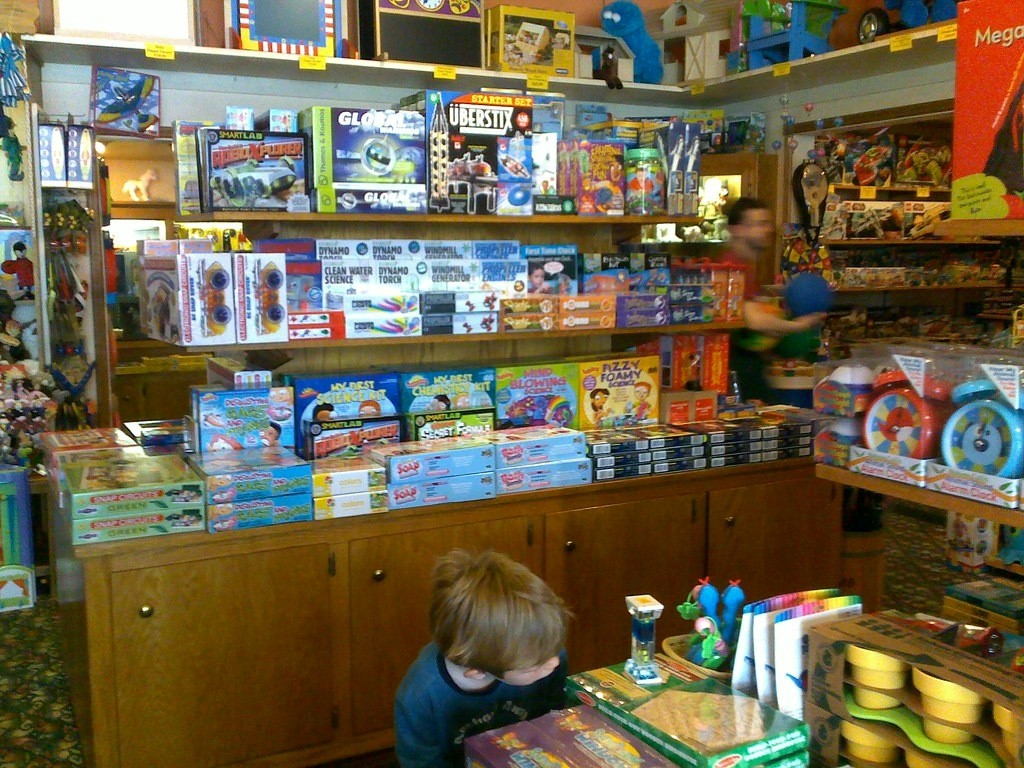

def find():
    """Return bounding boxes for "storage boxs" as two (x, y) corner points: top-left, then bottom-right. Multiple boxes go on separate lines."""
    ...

(925, 352), (1024, 509)
(941, 512), (1024, 636)
(313, 489), (389, 521)
(563, 654), (810, 768)
(205, 493), (313, 534)
(529, 703), (681, 768)
(803, 701), (976, 768)
(463, 721), (588, 768)
(196, 385), (295, 453)
(806, 613), (1024, 768)
(186, 445), (313, 505)
(304, 412), (407, 460)
(90, 0), (1024, 347)
(205, 356), (272, 385)
(362, 349), (840, 511)
(813, 353), (935, 487)
(308, 455), (387, 497)
(39, 428), (139, 468)
(60, 446), (206, 519)
(188, 382), (234, 450)
(289, 370), (399, 460)
(61, 507), (206, 546)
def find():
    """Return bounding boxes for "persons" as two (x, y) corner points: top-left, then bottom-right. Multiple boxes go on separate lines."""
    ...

(726, 197), (828, 407)
(393, 550), (578, 768)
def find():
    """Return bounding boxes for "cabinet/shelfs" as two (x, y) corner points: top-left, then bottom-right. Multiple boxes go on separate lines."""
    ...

(90, 126), (213, 427)
(51, 205), (845, 768)
(818, 183), (1006, 292)
